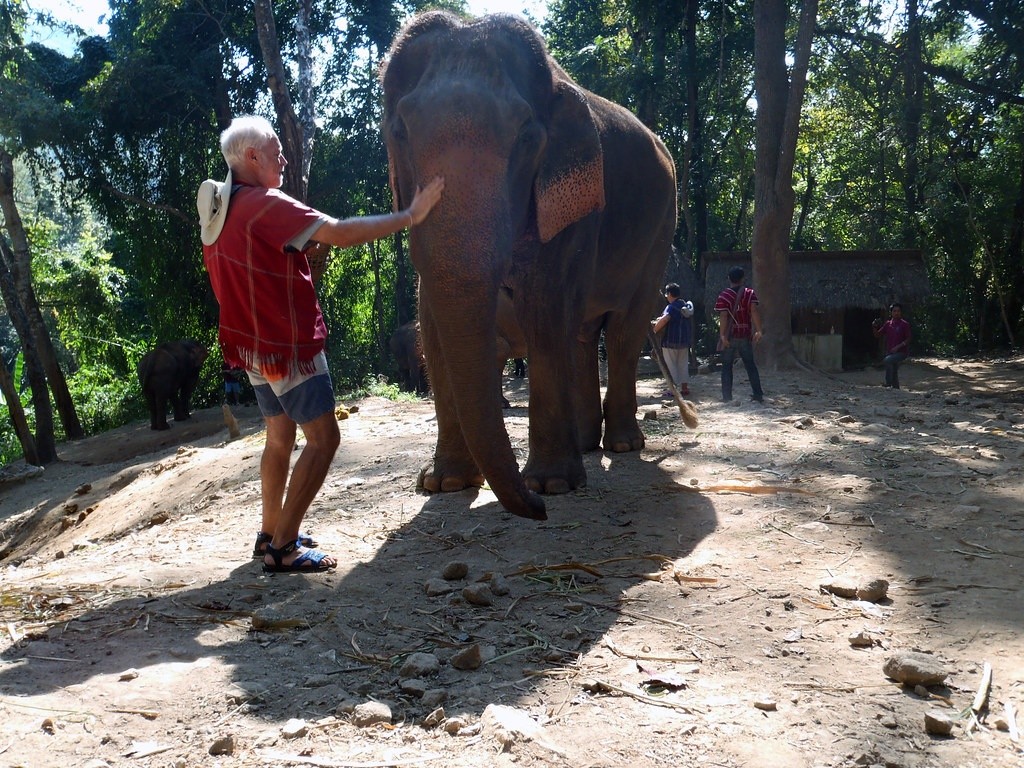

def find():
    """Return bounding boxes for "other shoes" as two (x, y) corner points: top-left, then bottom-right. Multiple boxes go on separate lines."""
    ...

(882, 383), (899, 389)
(680, 390), (689, 396)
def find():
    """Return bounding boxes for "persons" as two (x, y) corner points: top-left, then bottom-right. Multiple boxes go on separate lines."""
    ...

(221, 363), (240, 406)
(714, 266), (765, 403)
(651, 283), (692, 397)
(201, 114), (446, 573)
(872, 303), (911, 390)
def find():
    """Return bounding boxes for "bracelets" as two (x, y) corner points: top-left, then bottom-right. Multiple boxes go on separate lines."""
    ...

(405, 210), (414, 227)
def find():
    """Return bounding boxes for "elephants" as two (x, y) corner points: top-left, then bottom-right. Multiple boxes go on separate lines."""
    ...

(380, 8), (701, 521)
(137, 339), (209, 431)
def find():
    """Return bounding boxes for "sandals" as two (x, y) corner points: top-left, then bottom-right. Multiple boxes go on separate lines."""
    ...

(252, 532), (318, 559)
(262, 541), (337, 573)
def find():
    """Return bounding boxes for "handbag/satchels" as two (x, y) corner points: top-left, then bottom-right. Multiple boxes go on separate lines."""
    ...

(716, 323), (731, 352)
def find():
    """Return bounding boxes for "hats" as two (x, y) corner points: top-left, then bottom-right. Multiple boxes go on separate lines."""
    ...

(681, 300), (694, 318)
(197, 168), (232, 245)
